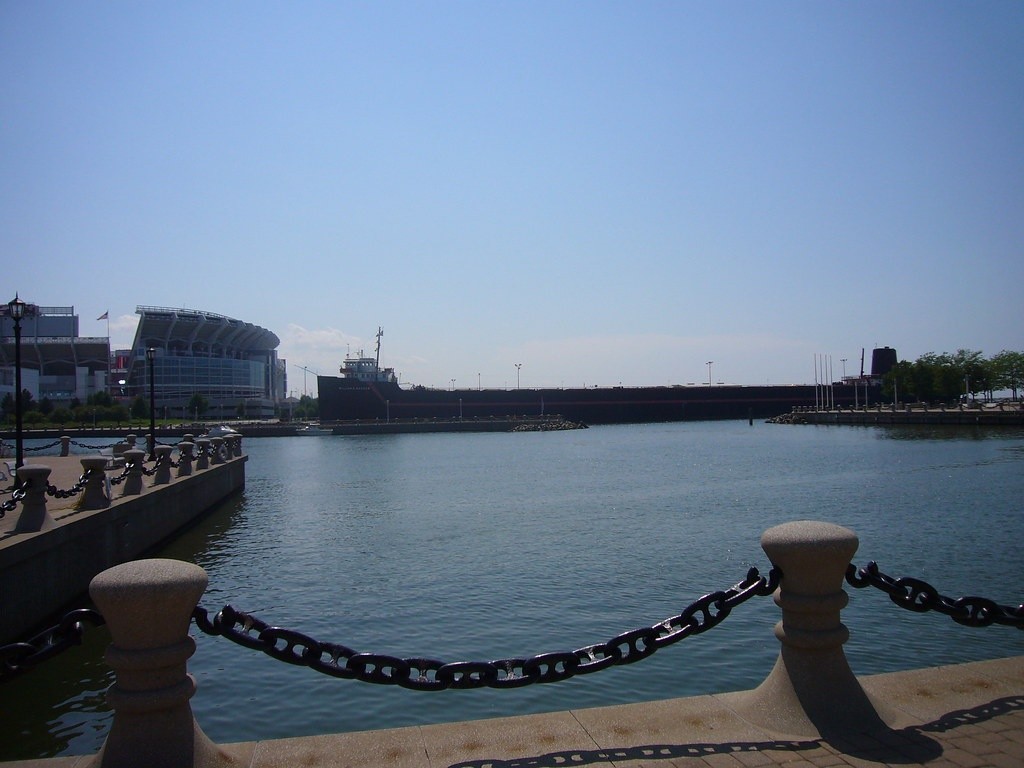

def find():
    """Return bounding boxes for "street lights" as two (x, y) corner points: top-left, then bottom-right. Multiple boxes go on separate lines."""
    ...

(705, 361), (714, 388)
(10, 291), (27, 495)
(145, 347), (157, 462)
(839, 358), (848, 382)
(514, 363), (522, 389)
(450, 378), (456, 393)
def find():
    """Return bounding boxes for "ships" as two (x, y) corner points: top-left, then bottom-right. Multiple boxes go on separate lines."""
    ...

(314, 326), (887, 423)
